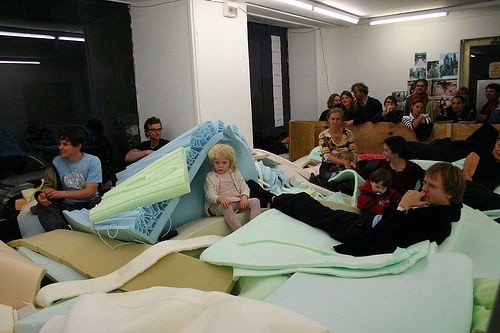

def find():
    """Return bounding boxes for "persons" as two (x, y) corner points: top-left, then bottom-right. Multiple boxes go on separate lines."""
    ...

(357, 168), (403, 214)
(202, 143), (261, 231)
(318, 78), (500, 211)
(35, 126), (103, 233)
(245, 160), (466, 257)
(122, 116), (170, 163)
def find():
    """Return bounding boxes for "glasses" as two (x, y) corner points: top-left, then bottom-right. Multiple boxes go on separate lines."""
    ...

(421, 181), (439, 191)
(151, 128), (164, 131)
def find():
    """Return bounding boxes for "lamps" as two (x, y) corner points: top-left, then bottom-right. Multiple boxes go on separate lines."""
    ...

(226, 0), (360, 30)
(369, 11), (449, 26)
